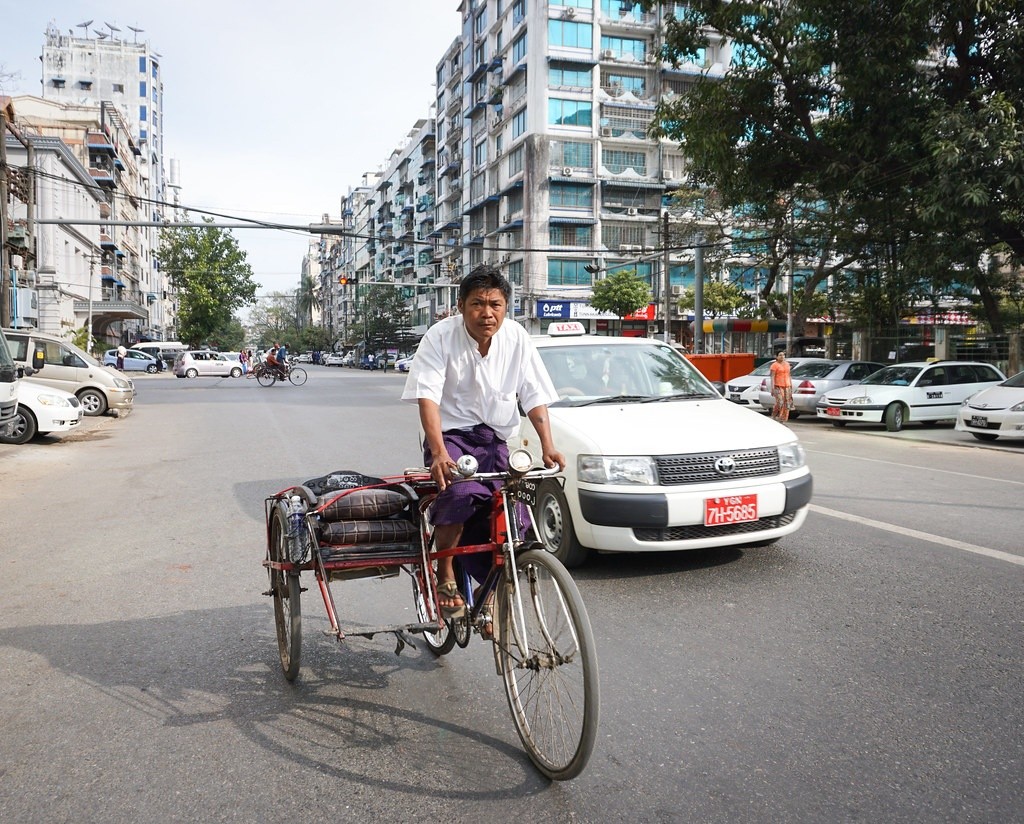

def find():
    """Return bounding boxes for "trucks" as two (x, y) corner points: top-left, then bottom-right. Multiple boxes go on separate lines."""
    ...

(0, 324), (41, 440)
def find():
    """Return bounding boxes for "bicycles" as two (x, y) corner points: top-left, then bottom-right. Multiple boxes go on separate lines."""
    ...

(257, 360), (308, 388)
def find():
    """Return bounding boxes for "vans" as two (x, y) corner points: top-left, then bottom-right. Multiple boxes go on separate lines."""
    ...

(3, 328), (138, 417)
(129, 341), (182, 358)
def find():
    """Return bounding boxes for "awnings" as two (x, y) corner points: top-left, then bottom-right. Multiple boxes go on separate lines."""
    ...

(101, 243), (118, 250)
(115, 160), (125, 171)
(102, 275), (116, 282)
(147, 294), (157, 300)
(116, 250), (125, 257)
(117, 281), (125, 287)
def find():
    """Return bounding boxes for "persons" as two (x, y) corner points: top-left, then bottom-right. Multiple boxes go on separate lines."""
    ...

(156, 347), (162, 373)
(770, 351), (795, 425)
(115, 344), (126, 369)
(368, 353), (374, 371)
(239, 341), (291, 382)
(400, 264), (566, 636)
(349, 353), (352, 368)
(312, 351), (320, 365)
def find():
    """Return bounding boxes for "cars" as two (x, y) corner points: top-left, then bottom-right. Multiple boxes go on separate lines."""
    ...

(287, 350), (397, 367)
(954, 370), (1024, 443)
(723, 358), (830, 415)
(534, 332), (813, 572)
(172, 350), (243, 378)
(103, 349), (168, 374)
(394, 353), (416, 372)
(2, 377), (84, 445)
(815, 357), (1008, 431)
(758, 359), (888, 419)
(221, 351), (241, 362)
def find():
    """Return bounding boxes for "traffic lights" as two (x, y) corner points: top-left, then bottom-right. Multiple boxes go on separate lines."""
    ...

(340, 278), (359, 285)
(584, 264), (600, 273)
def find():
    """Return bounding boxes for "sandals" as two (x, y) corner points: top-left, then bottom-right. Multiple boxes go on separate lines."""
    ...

(471, 592), (494, 641)
(434, 577), (464, 613)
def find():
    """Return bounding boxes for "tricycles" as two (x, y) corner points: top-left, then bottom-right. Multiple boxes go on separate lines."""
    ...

(263, 447), (602, 783)
